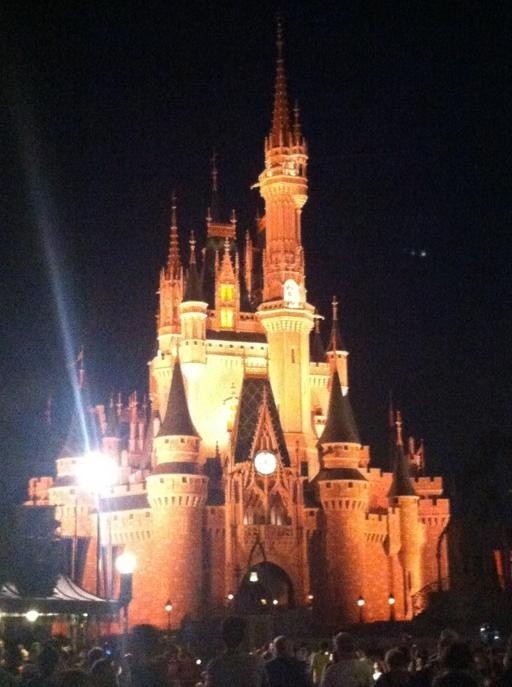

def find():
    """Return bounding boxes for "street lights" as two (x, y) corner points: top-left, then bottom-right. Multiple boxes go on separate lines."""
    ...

(115, 546), (137, 636)
(355, 594), (367, 624)
(163, 598), (174, 636)
(71, 444), (129, 598)
(223, 590), (316, 619)
(387, 592), (397, 623)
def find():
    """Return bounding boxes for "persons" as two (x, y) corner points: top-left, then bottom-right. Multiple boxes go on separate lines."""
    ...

(1, 614), (512, 687)
